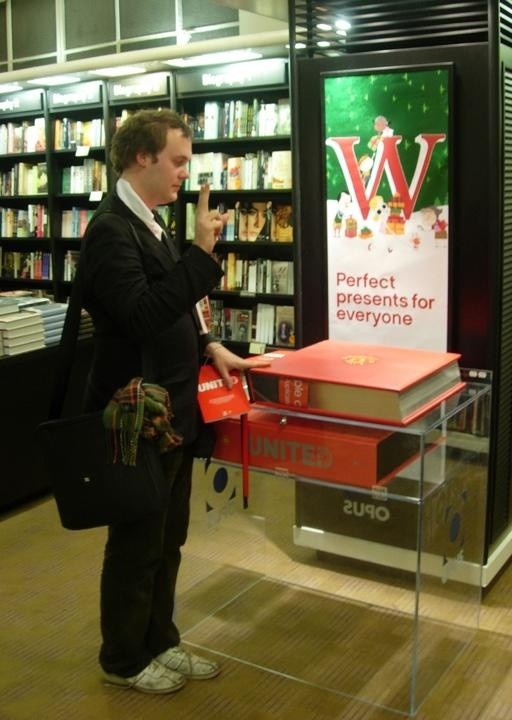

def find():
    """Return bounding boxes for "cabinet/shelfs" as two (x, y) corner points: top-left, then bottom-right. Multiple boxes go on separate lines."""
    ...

(172, 371), (493, 717)
(2, 56), (297, 353)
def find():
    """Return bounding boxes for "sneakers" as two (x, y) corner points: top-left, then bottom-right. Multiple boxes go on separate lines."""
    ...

(154, 644), (222, 680)
(102, 658), (188, 695)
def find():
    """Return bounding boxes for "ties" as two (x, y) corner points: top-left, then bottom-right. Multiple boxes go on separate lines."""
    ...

(160, 231), (175, 257)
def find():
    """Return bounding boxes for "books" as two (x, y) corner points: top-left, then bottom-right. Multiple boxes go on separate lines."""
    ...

(45, 336), (62, 345)
(0, 290), (33, 298)
(190, 363), (251, 425)
(153, 206), (176, 241)
(236, 260), (240, 287)
(3, 174), (6, 196)
(249, 258), (295, 296)
(204, 103), (223, 140)
(0, 176), (2, 196)
(71, 166), (84, 194)
(61, 207), (97, 238)
(42, 313), (66, 324)
(0, 246), (53, 280)
(0, 332), (44, 347)
(0, 298), (50, 311)
(222, 98), (290, 140)
(64, 249), (80, 281)
(19, 163), (48, 195)
(0, 312), (43, 331)
(55, 120), (60, 151)
(42, 321), (65, 331)
(27, 288), (48, 298)
(43, 328), (64, 337)
(228, 253), (236, 291)
(11, 168), (14, 196)
(62, 168), (71, 194)
(256, 303), (295, 348)
(20, 303), (68, 317)
(1, 204), (50, 237)
(14, 165), (18, 196)
(244, 260), (247, 290)
(70, 119), (106, 151)
(6, 172), (11, 197)
(102, 165), (107, 192)
(114, 107), (170, 129)
(94, 161), (102, 191)
(61, 124), (63, 150)
(0, 324), (44, 339)
(210, 251), (227, 290)
(0, 117), (47, 155)
(248, 340), (468, 425)
(63, 118), (69, 151)
(192, 295), (212, 335)
(210, 299), (253, 342)
(0, 340), (48, 357)
(212, 405), (449, 490)
(186, 202), (195, 239)
(185, 151), (292, 191)
(216, 201), (294, 242)
(0, 299), (21, 316)
(240, 260), (243, 288)
(84, 159), (93, 194)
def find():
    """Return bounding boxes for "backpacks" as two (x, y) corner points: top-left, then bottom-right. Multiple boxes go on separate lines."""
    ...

(51, 212), (140, 530)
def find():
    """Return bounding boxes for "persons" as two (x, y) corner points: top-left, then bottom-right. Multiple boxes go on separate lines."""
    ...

(77, 109), (271, 694)
(279, 322), (288, 340)
(270, 205), (292, 241)
(247, 199), (267, 242)
(237, 324), (247, 340)
(236, 200), (248, 242)
